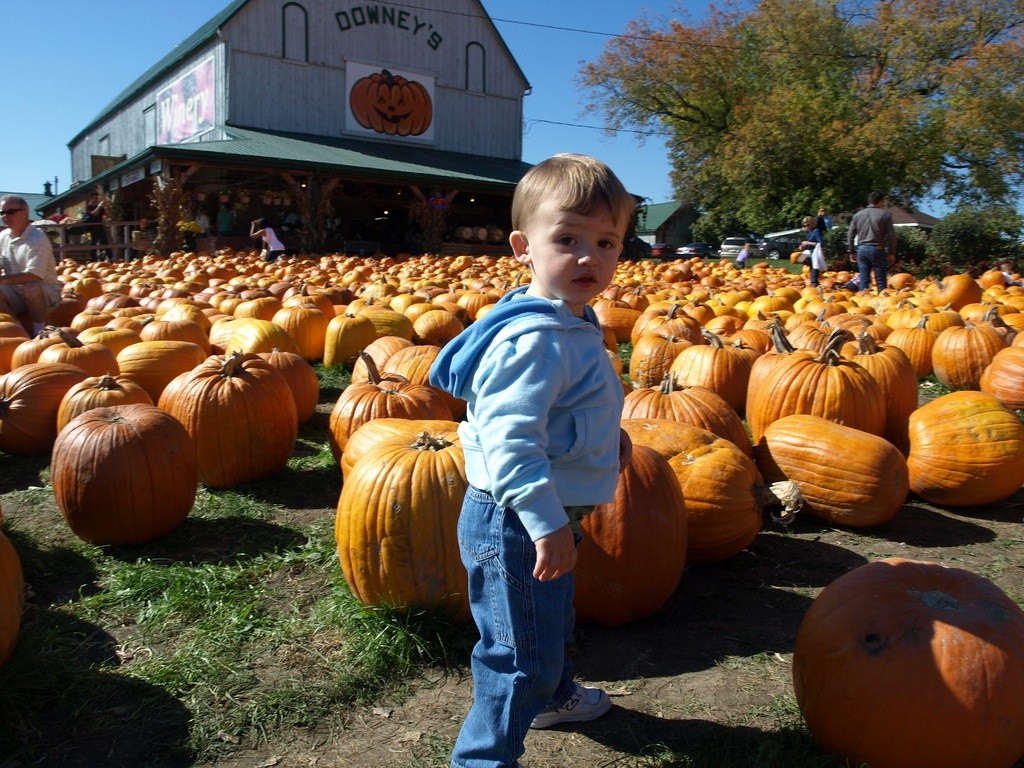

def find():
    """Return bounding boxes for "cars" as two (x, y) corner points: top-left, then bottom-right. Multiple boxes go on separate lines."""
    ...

(759, 234), (809, 261)
(649, 240), (720, 260)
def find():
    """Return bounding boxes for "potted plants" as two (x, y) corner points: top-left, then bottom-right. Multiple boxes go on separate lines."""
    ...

(193, 186), (208, 202)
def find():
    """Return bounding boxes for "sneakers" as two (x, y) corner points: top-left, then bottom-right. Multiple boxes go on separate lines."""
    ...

(530, 684), (611, 728)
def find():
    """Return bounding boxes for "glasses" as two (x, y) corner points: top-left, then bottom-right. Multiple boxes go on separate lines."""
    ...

(0, 208), (26, 217)
(802, 224), (811, 228)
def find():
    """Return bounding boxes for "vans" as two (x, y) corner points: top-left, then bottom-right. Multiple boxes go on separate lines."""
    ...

(718, 236), (748, 258)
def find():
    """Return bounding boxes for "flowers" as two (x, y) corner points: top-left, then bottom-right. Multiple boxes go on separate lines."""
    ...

(237, 186), (252, 197)
(215, 189), (232, 196)
(176, 220), (202, 252)
(260, 190), (291, 199)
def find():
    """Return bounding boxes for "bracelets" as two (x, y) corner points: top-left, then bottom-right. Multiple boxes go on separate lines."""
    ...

(98, 203), (102, 208)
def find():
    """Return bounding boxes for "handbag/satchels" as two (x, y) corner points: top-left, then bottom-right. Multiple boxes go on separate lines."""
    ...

(811, 244), (828, 270)
(259, 249), (270, 261)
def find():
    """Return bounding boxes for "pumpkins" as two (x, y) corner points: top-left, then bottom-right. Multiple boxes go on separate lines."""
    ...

(0, 247), (1023, 768)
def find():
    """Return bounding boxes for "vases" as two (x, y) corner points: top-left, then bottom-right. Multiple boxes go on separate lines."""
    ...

(262, 196), (291, 206)
(218, 196), (230, 202)
(239, 196), (250, 204)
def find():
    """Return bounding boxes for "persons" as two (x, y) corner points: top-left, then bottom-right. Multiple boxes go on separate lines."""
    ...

(428, 151), (637, 768)
(281, 207), (304, 230)
(817, 207), (832, 230)
(195, 206), (212, 238)
(847, 191), (896, 295)
(888, 255), (1011, 275)
(799, 216), (823, 287)
(249, 217), (286, 263)
(735, 243), (752, 269)
(86, 191), (115, 264)
(216, 203), (237, 241)
(0, 193), (61, 338)
